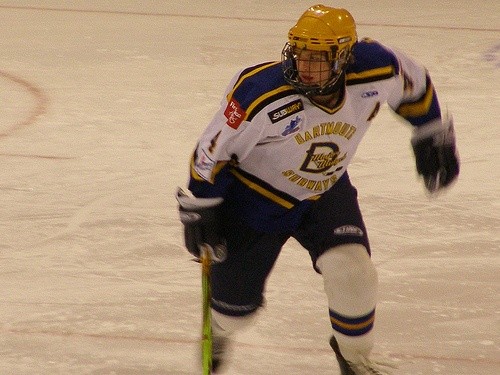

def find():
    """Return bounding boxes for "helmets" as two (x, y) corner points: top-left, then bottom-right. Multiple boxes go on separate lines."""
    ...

(288, 4), (357, 87)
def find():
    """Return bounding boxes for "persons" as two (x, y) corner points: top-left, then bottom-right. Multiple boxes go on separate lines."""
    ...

(176, 4), (459, 375)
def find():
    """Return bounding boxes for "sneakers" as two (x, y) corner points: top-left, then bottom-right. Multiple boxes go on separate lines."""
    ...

(200, 334), (230, 372)
(329, 335), (386, 375)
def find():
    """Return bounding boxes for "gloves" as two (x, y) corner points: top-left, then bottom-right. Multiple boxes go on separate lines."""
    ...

(175, 185), (232, 265)
(411, 113), (460, 193)
